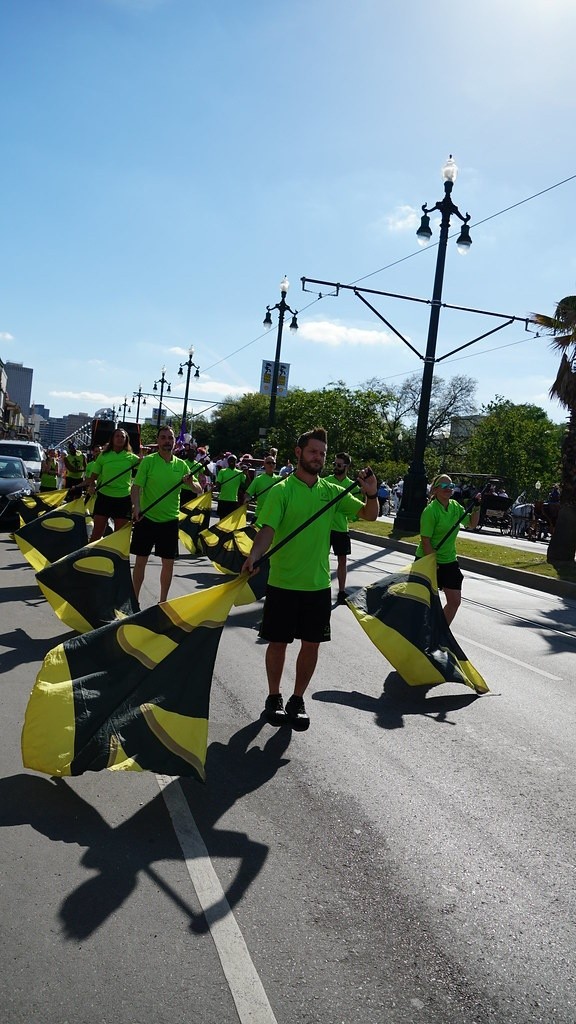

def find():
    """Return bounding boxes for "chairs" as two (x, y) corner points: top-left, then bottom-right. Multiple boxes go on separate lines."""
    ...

(4, 463), (17, 474)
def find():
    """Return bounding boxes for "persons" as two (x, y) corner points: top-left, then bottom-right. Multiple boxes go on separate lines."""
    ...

(323, 452), (364, 603)
(39, 443), (94, 502)
(130, 424), (202, 607)
(216, 455), (252, 520)
(242, 456), (286, 531)
(485, 482), (511, 499)
(240, 429), (380, 734)
(376, 474), (404, 516)
(175, 441), (296, 502)
(413, 473), (481, 629)
(80, 428), (142, 543)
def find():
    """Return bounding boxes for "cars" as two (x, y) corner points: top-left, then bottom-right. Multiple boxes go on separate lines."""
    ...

(0, 455), (37, 500)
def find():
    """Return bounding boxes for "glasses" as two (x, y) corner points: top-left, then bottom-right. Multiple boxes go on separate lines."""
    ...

(265, 461), (277, 465)
(435, 482), (455, 489)
(332, 462), (347, 467)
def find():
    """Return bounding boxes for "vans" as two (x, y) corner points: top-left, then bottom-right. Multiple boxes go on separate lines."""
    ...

(0, 438), (47, 493)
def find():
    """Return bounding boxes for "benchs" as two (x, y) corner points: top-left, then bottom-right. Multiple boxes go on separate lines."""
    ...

(482, 494), (512, 511)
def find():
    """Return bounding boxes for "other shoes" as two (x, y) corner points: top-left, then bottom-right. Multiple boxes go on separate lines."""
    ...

(338, 593), (349, 605)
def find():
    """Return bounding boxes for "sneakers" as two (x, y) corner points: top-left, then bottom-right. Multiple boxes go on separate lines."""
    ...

(265, 696), (286, 720)
(285, 698), (309, 725)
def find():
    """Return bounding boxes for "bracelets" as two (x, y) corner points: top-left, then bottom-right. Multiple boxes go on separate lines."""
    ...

(366, 493), (379, 499)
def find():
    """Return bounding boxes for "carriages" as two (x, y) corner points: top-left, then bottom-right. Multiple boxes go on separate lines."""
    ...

(380, 472), (561, 543)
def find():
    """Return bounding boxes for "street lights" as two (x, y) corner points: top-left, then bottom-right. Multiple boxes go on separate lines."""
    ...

(53, 402), (118, 453)
(119, 395), (132, 422)
(395, 433), (404, 464)
(177, 344), (201, 443)
(132, 381), (147, 423)
(262, 274), (299, 459)
(152, 364), (172, 429)
(535, 480), (542, 500)
(392, 153), (474, 533)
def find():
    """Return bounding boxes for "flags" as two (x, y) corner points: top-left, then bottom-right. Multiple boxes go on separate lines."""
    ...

(16, 488), (142, 632)
(347, 553), (491, 694)
(180, 491), (271, 606)
(21, 573), (253, 781)
